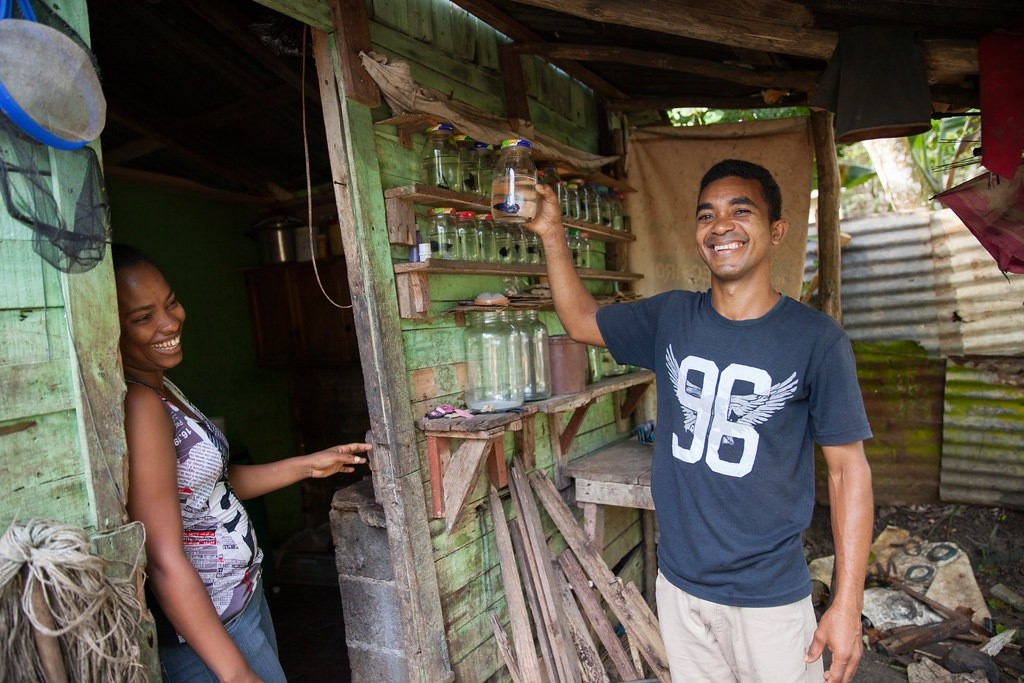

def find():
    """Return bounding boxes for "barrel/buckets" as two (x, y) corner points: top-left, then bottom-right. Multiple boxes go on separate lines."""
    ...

(549, 335), (588, 395)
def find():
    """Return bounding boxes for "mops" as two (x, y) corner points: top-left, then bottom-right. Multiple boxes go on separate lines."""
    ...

(0, 508), (151, 683)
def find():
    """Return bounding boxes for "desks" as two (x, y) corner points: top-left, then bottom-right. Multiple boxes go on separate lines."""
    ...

(567, 431), (656, 658)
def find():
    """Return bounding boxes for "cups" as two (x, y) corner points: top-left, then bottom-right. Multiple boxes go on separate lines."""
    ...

(294, 227), (318, 262)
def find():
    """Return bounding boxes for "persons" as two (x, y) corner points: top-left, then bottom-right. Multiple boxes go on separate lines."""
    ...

(495, 160), (875, 683)
(113, 255), (373, 683)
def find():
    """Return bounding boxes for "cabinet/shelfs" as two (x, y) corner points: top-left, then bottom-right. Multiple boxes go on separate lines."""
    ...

(247, 255), (366, 369)
(385, 183), (645, 319)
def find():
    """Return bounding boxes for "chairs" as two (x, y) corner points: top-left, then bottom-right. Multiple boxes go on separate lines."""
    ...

(278, 550), (346, 662)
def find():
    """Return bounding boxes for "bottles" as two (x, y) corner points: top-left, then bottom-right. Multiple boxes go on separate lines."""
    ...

(493, 222), (514, 264)
(476, 214), (497, 263)
(425, 208), (459, 260)
(587, 344), (639, 383)
(454, 134), (501, 200)
(526, 232), (540, 265)
(455, 211), (479, 261)
(491, 139), (537, 224)
(318, 218), (344, 258)
(421, 125), (462, 193)
(269, 219), (295, 265)
(537, 165), (624, 231)
(409, 224), (421, 262)
(512, 310), (552, 400)
(511, 224), (530, 265)
(580, 233), (592, 268)
(463, 311), (524, 411)
(539, 237), (546, 264)
(564, 229), (581, 267)
